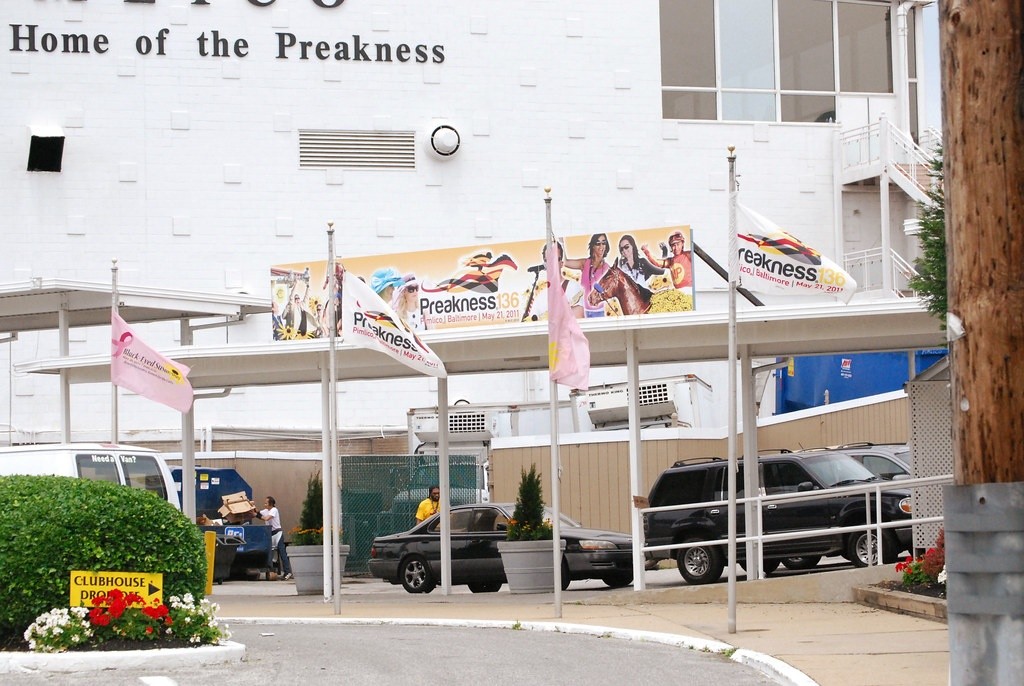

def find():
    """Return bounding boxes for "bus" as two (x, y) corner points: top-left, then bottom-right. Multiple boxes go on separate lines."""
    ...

(0, 441), (183, 515)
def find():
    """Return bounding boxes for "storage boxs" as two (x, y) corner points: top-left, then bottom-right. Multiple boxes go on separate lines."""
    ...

(218, 491), (256, 523)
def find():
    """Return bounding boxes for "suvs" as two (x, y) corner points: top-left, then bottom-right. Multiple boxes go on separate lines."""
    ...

(642, 448), (915, 586)
(778, 440), (927, 572)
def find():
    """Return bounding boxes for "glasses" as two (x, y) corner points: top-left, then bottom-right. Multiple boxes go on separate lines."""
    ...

(669, 234), (681, 240)
(407, 285), (419, 293)
(620, 244), (630, 252)
(594, 240), (607, 245)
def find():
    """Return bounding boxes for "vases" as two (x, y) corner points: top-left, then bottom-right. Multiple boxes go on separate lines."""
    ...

(286, 545), (350, 595)
(497, 540), (567, 594)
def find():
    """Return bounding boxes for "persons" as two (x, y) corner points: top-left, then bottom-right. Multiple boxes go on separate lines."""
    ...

(415, 485), (440, 526)
(521, 231), (692, 323)
(249, 496), (294, 581)
(272, 267), (435, 341)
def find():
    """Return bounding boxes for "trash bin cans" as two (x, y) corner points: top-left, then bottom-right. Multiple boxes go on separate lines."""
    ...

(213, 534), (247, 584)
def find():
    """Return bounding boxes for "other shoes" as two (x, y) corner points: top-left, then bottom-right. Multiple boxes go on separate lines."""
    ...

(281, 573), (294, 580)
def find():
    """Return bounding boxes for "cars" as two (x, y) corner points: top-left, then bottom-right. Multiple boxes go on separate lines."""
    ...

(366, 501), (634, 594)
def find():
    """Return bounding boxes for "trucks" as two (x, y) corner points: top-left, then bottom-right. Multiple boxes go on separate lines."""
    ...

(381, 459), (490, 532)
(144, 464), (276, 582)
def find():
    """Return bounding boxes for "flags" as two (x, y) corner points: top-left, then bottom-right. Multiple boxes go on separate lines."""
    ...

(736, 193), (858, 305)
(111, 308), (193, 415)
(547, 241), (590, 391)
(342, 267), (447, 379)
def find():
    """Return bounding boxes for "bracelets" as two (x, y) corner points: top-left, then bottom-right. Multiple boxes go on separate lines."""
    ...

(255, 513), (262, 520)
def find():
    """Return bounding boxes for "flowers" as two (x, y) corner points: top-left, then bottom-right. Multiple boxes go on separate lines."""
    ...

(896, 548), (935, 587)
(289, 469), (344, 545)
(23, 590), (232, 653)
(506, 461), (554, 541)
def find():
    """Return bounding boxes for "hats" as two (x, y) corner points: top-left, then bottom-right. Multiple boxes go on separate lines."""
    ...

(668, 231), (684, 245)
(371, 268), (406, 294)
(403, 273), (419, 288)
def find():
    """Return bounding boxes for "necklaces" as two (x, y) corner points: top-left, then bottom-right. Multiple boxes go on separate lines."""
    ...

(589, 258), (602, 278)
(431, 498), (439, 513)
